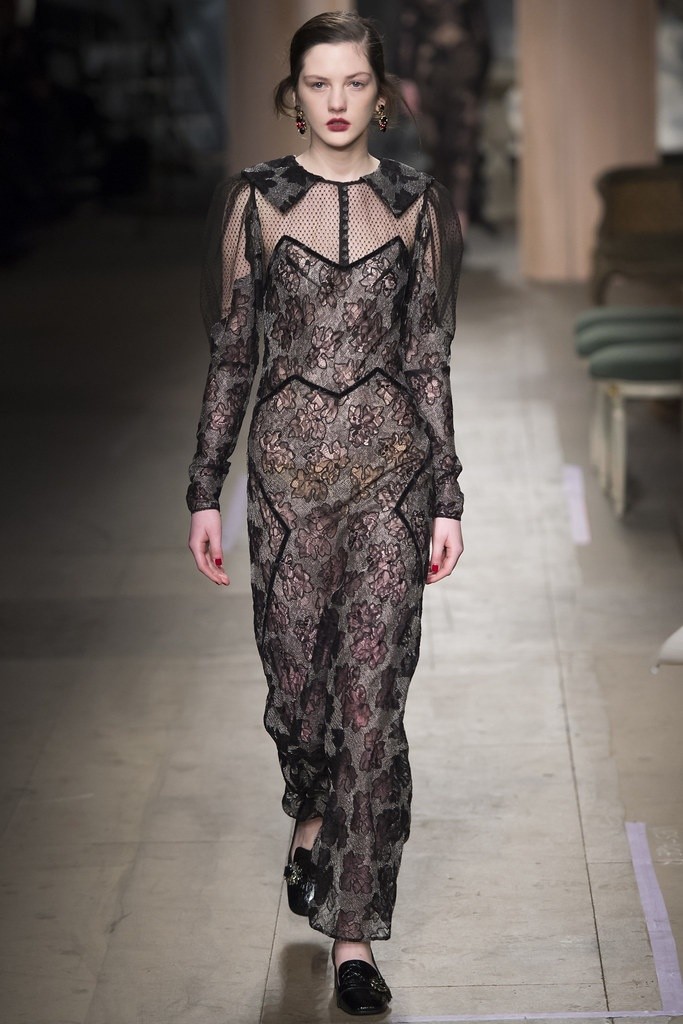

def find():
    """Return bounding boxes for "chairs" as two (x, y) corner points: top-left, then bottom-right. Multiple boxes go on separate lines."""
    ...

(591, 165), (683, 306)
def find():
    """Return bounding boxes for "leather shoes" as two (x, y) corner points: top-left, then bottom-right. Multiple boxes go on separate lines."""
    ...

(282, 819), (329, 916)
(331, 940), (392, 1015)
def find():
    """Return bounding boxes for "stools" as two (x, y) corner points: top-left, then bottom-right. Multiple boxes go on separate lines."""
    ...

(574, 306), (683, 474)
(576, 322), (683, 494)
(589, 341), (683, 516)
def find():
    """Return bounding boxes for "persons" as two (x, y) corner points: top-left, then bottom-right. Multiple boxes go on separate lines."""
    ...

(185, 9), (465, 1015)
